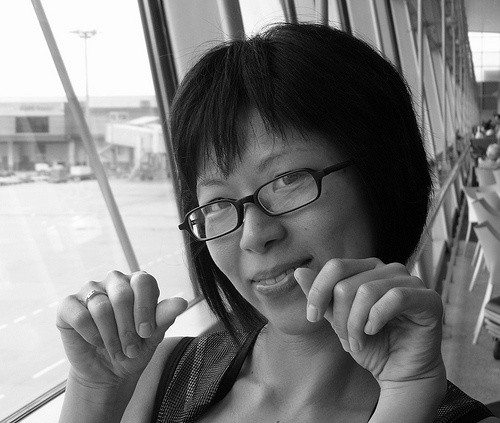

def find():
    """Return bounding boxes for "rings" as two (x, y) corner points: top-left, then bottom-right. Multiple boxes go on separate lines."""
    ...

(83, 290), (108, 308)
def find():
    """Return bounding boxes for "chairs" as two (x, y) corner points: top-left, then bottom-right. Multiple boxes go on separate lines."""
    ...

(461, 125), (500, 345)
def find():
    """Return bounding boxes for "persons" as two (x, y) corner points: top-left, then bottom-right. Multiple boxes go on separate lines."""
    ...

(54, 19), (499, 423)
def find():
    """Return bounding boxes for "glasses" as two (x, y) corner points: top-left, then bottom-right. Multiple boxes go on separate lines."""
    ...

(180, 160), (354, 241)
